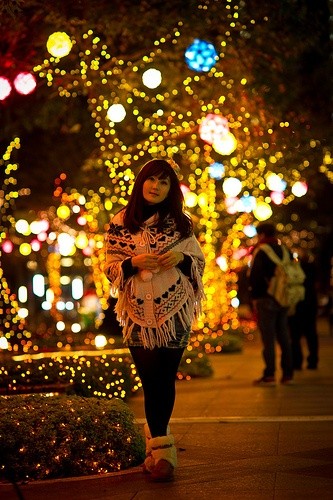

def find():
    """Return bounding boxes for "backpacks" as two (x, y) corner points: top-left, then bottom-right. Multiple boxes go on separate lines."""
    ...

(260, 244), (305, 307)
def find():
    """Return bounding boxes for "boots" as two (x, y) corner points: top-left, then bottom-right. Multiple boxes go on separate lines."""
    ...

(149, 434), (176, 482)
(145, 436), (154, 474)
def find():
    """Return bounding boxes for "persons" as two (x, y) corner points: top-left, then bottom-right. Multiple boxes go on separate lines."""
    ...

(103, 160), (205, 479)
(249, 223), (319, 387)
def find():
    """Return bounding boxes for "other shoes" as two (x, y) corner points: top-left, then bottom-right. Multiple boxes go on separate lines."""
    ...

(253, 377), (275, 386)
(280, 378), (294, 384)
